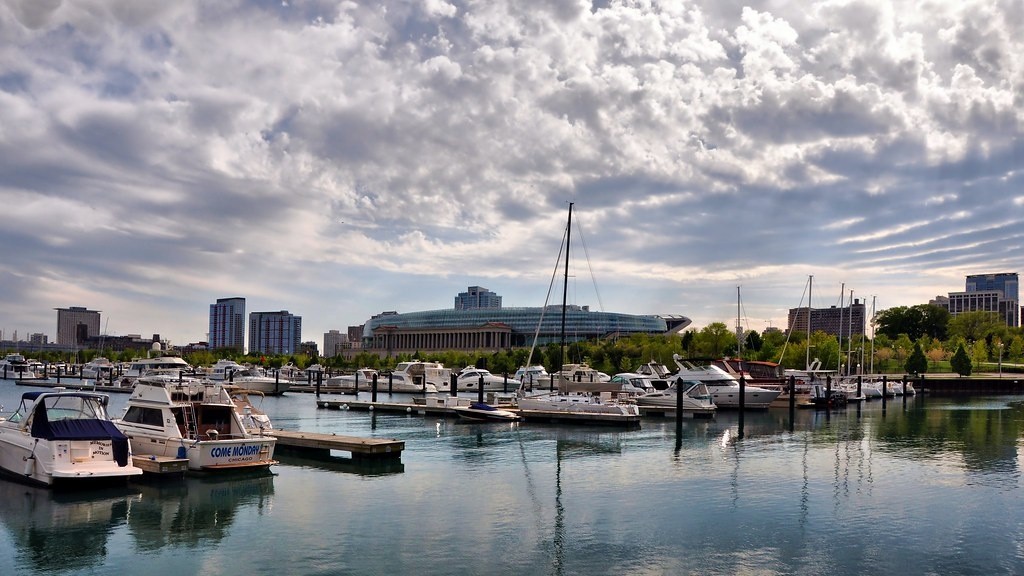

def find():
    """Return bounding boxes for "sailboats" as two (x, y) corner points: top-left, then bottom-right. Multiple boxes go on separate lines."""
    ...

(810, 282), (916, 400)
(512, 199), (641, 421)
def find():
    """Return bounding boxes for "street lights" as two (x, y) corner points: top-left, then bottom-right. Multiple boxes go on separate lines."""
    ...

(997, 343), (1004, 378)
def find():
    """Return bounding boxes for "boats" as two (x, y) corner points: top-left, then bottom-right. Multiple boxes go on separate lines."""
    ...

(0, 355), (36, 379)
(78, 358), (115, 378)
(514, 365), (548, 386)
(109, 370), (280, 476)
(537, 364), (604, 389)
(284, 364), (329, 381)
(123, 356), (205, 383)
(438, 365), (521, 392)
(615, 363), (671, 382)
(0, 387), (143, 488)
(366, 359), (457, 393)
(220, 368), (293, 395)
(332, 368), (381, 391)
(454, 402), (521, 421)
(634, 354), (782, 409)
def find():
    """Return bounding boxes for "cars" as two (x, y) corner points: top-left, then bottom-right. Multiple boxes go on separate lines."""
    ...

(344, 370), (354, 375)
(333, 369), (344, 374)
(598, 372), (610, 381)
